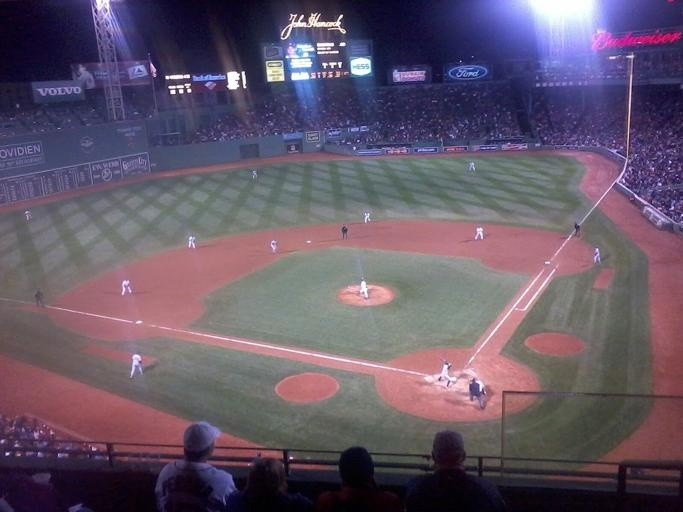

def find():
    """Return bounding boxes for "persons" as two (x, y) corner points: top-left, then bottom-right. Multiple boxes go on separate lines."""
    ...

(317, 446), (405, 511)
(593, 246), (601, 264)
(271, 240), (278, 252)
(1, 48), (683, 239)
(1, 413), (69, 457)
(439, 361), (453, 387)
(130, 352), (144, 379)
(228, 455), (313, 510)
(156, 421), (235, 510)
(400, 427), (504, 511)
(360, 277), (369, 299)
(35, 290), (44, 307)
(188, 235), (196, 249)
(468, 375), (488, 410)
(121, 279), (132, 296)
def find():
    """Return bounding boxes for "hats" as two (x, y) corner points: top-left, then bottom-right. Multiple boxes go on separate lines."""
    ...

(184, 424), (220, 450)
(432, 432), (464, 458)
